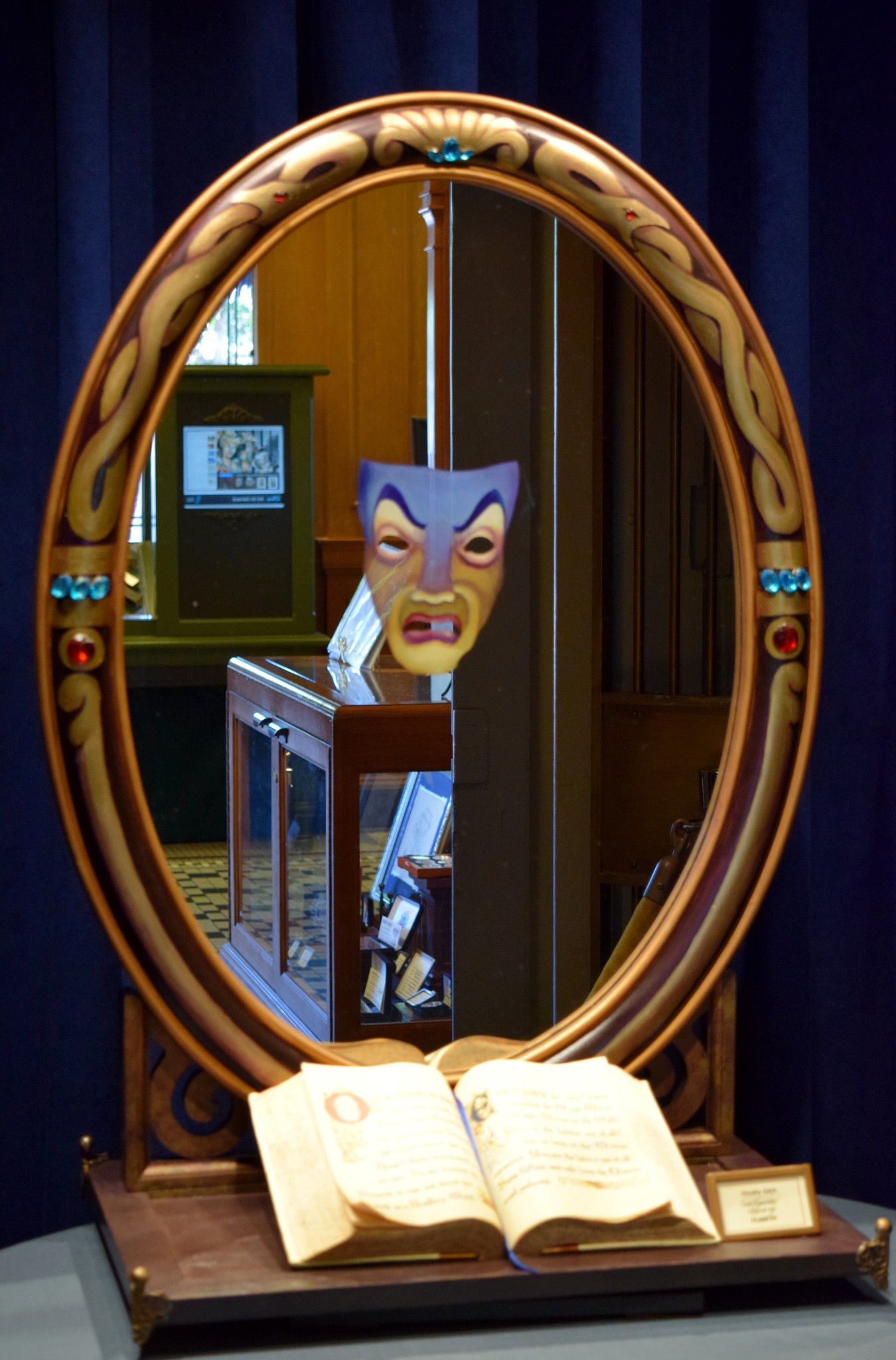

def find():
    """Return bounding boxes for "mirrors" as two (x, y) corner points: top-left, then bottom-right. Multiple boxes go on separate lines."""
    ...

(36, 91), (824, 1099)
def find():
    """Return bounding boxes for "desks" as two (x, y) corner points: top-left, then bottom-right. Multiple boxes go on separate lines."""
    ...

(120, 635), (334, 841)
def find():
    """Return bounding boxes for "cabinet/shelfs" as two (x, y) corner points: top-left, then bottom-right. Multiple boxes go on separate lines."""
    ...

(220, 657), (453, 1046)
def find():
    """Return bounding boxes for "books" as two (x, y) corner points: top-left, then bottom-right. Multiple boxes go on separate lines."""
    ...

(247, 1056), (721, 1268)
